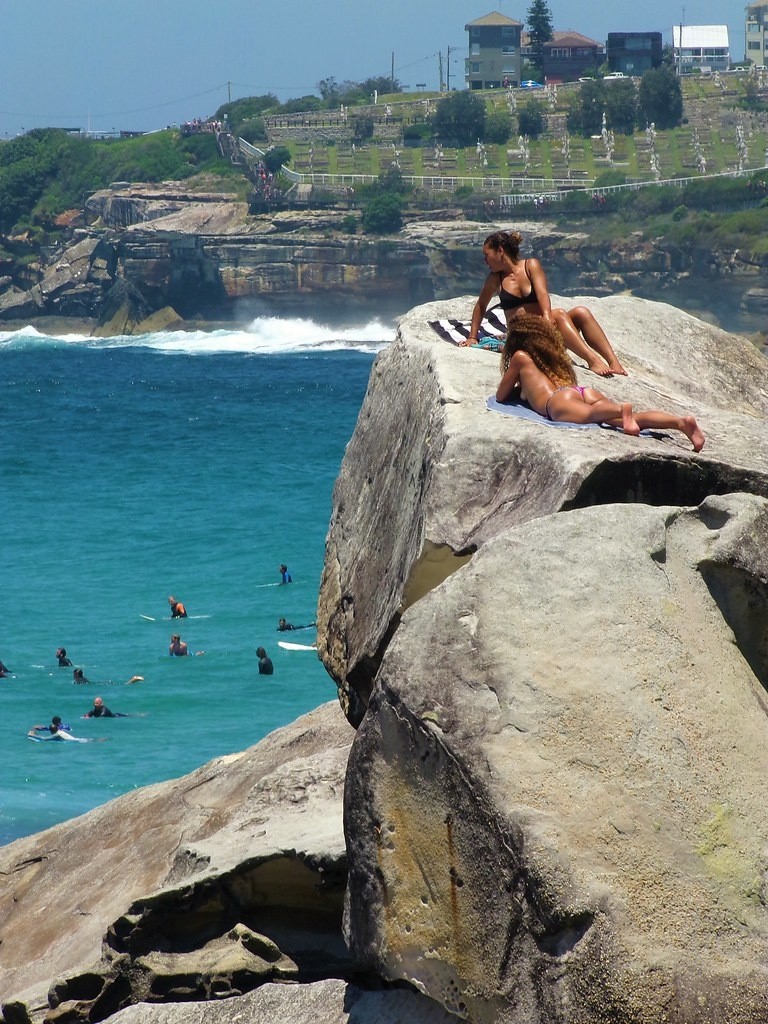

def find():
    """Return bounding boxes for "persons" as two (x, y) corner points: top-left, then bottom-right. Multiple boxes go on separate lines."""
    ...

(172, 117), (282, 201)
(276, 617), (316, 632)
(458, 231), (629, 377)
(280, 565), (292, 584)
(257, 647), (273, 675)
(55, 648), (72, 667)
(746, 180), (766, 194)
(72, 668), (144, 686)
(484, 193), (605, 210)
(28, 725), (111, 744)
(169, 633), (204, 656)
(82, 698), (147, 718)
(34, 716), (73, 731)
(496, 313), (706, 453)
(168, 596), (187, 618)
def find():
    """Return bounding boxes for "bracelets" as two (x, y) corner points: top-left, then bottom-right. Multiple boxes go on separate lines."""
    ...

(468, 336), (479, 341)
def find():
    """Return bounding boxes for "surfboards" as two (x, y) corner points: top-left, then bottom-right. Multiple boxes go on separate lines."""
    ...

(255, 582), (281, 588)
(277, 640), (318, 651)
(27, 735), (94, 743)
(158, 614), (213, 619)
